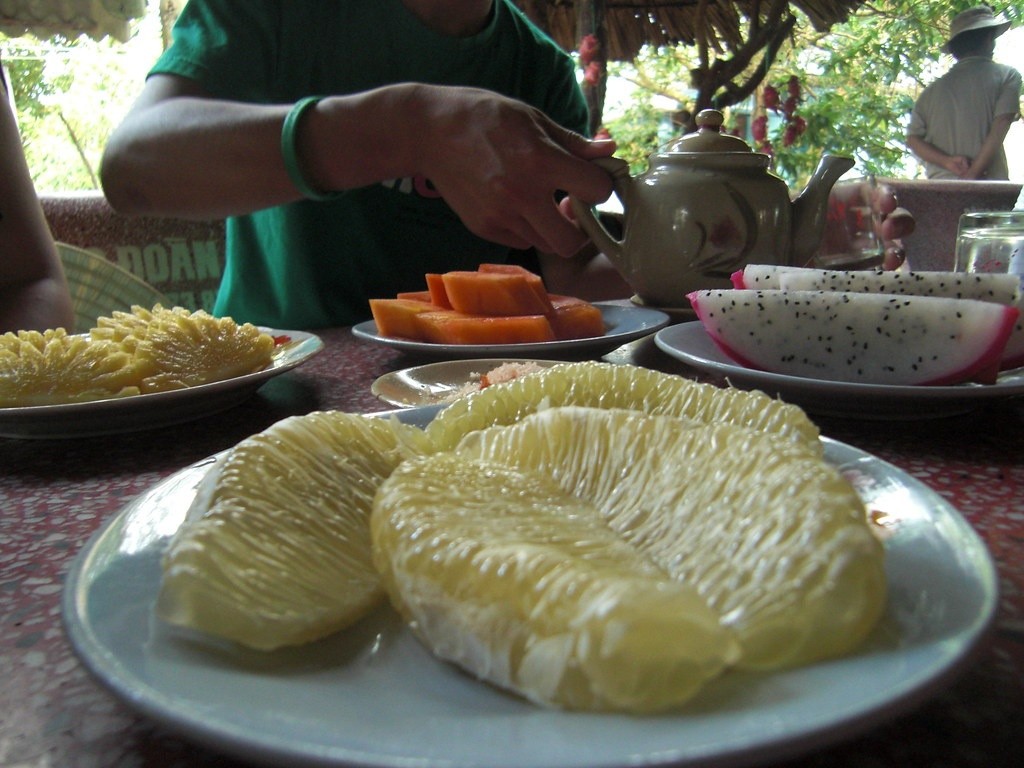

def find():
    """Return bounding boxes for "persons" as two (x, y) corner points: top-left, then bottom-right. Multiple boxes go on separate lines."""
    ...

(906, 6), (1021, 180)
(100, 0), (915, 332)
(0, 61), (76, 335)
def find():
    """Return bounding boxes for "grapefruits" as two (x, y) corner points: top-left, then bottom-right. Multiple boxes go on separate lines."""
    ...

(158, 361), (887, 713)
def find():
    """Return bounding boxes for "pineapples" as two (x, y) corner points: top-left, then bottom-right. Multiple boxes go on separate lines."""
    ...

(0, 302), (274, 408)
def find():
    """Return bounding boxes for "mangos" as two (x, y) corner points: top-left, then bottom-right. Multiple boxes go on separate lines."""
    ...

(0, 0), (147, 44)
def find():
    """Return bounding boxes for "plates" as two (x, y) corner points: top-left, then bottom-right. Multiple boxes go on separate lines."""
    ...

(654, 320), (1024, 421)
(351, 304), (670, 362)
(0, 325), (326, 440)
(371, 359), (576, 410)
(60, 403), (1000, 768)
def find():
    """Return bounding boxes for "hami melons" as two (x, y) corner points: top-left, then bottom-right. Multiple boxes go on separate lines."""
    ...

(369, 263), (605, 345)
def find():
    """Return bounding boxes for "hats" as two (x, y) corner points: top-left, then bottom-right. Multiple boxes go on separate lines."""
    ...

(941, 5), (1012, 54)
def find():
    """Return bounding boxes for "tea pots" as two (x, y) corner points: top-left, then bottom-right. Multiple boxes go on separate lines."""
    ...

(569, 108), (856, 306)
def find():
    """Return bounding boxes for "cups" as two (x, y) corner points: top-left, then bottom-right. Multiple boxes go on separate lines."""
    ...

(954, 209), (1024, 309)
(814, 175), (885, 275)
(876, 177), (1024, 272)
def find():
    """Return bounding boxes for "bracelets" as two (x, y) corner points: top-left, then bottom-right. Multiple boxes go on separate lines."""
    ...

(280, 96), (346, 203)
